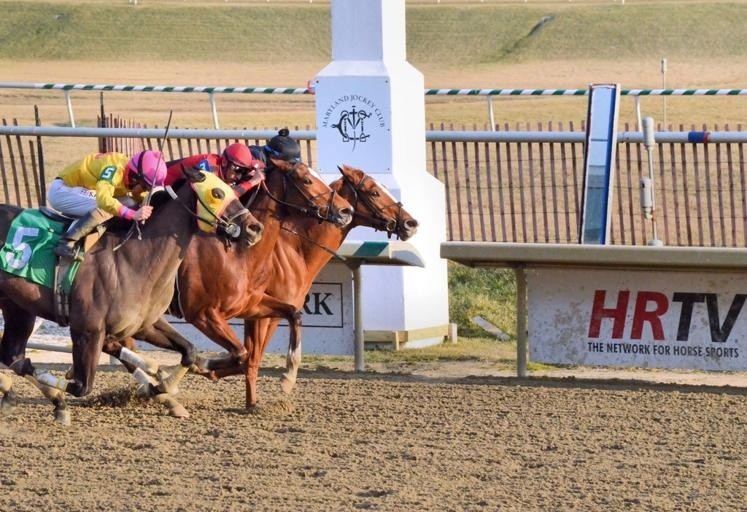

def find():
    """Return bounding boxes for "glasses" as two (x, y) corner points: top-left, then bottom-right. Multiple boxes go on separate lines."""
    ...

(138, 150), (150, 192)
(268, 142), (301, 164)
(224, 149), (248, 175)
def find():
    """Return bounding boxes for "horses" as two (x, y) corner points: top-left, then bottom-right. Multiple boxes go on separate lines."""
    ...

(64, 156), (355, 418)
(1, 164), (265, 425)
(244, 164), (419, 414)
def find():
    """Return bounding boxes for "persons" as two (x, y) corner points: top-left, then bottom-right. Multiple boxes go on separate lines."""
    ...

(163, 143), (267, 203)
(249, 133), (304, 167)
(46, 149), (167, 262)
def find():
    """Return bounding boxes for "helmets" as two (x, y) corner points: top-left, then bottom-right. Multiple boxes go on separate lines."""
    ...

(223, 144), (253, 169)
(267, 129), (300, 160)
(127, 150), (168, 189)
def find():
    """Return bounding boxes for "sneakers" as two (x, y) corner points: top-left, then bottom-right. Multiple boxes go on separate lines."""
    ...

(52, 241), (84, 262)
